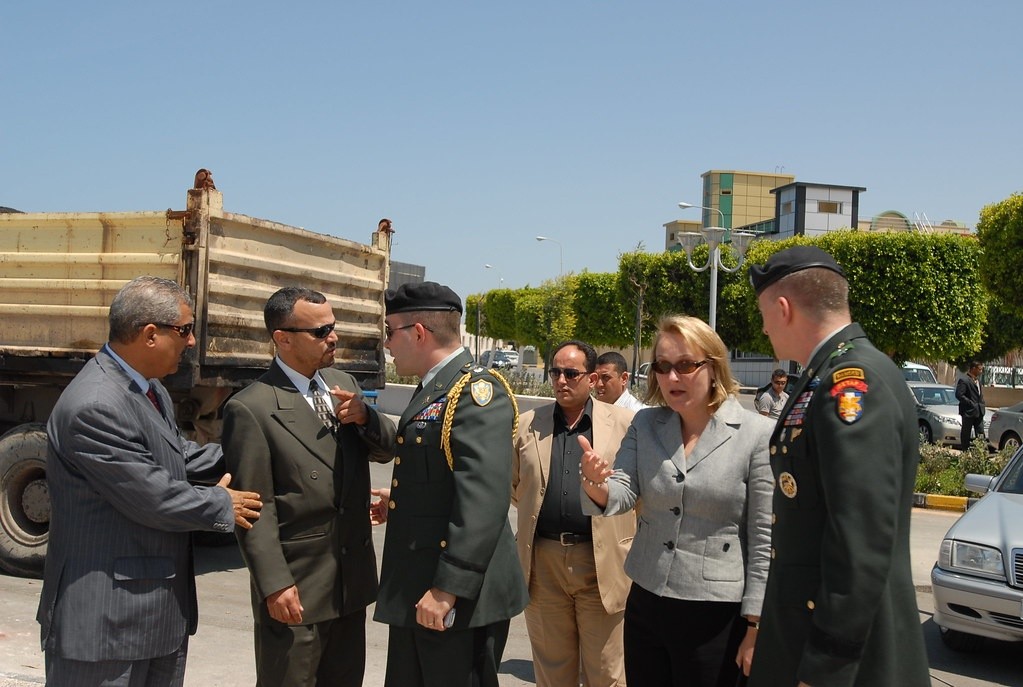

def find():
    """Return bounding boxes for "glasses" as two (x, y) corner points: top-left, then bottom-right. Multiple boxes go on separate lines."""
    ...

(773, 381), (787, 386)
(548, 367), (592, 380)
(650, 358), (710, 374)
(271, 322), (337, 338)
(386, 325), (434, 340)
(137, 320), (194, 337)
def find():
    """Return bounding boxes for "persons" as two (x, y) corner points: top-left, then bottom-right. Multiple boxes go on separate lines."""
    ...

(750, 245), (933, 687)
(221, 285), (398, 687)
(758, 368), (790, 421)
(510, 340), (640, 687)
(369, 282), (531, 687)
(578, 315), (777, 687)
(36, 277), (263, 687)
(593, 352), (651, 414)
(955, 360), (987, 450)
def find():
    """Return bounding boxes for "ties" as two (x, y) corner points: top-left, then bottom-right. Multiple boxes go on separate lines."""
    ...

(410, 380), (422, 404)
(147, 389), (161, 413)
(310, 380), (341, 446)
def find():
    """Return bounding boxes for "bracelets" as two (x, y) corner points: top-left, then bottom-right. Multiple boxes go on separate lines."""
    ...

(578, 462), (608, 487)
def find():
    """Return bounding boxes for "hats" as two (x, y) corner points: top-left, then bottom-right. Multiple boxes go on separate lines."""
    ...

(384, 281), (462, 314)
(750, 246), (846, 291)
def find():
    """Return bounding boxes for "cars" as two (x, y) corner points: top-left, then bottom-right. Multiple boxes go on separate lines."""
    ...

(754, 373), (801, 410)
(627, 361), (650, 391)
(905, 381), (994, 447)
(480, 351), (511, 372)
(931, 444), (1023, 656)
(989, 401), (1023, 452)
(501, 351), (520, 370)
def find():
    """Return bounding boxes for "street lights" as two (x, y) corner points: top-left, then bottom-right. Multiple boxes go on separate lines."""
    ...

(536, 236), (563, 275)
(677, 201), (725, 245)
(676, 227), (756, 330)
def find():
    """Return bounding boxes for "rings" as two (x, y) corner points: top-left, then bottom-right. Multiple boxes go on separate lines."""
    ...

(428, 623), (434, 626)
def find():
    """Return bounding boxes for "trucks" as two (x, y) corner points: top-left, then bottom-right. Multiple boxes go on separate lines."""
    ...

(1, 171), (396, 576)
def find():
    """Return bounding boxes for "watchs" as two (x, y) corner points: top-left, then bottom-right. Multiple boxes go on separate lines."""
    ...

(747, 621), (760, 630)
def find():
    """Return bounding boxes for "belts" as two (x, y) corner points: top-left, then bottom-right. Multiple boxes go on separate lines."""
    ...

(535, 529), (591, 547)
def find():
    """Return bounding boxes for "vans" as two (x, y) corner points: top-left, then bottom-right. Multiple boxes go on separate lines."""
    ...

(897, 363), (953, 402)
